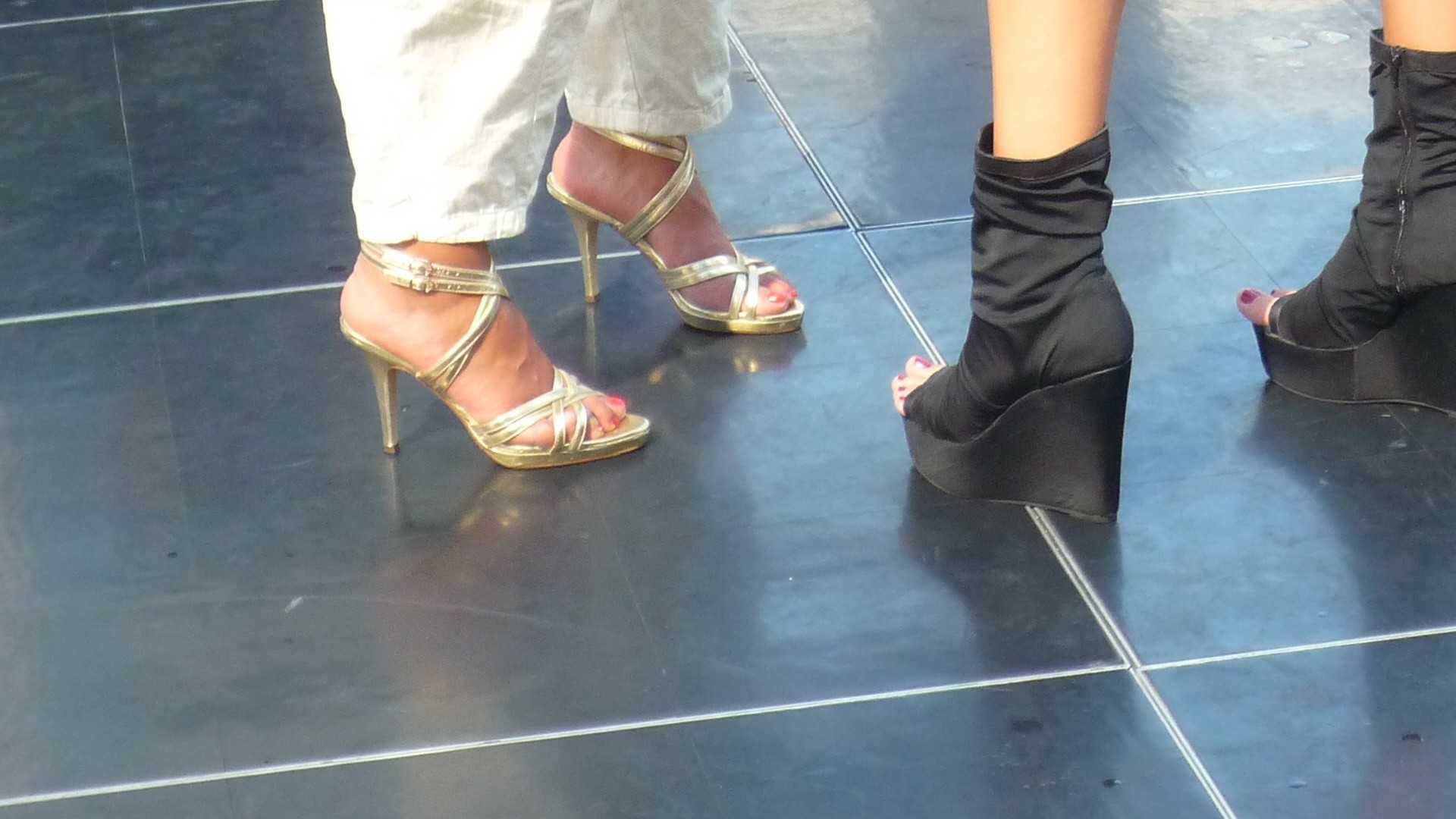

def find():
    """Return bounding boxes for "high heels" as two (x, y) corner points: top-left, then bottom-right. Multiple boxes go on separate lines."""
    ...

(545, 119), (803, 335)
(340, 239), (650, 468)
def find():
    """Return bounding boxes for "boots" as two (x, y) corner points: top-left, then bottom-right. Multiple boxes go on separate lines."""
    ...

(902, 121), (1135, 523)
(1251, 27), (1456, 415)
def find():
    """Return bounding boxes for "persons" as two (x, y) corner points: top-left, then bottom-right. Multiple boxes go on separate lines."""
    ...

(321, 0), (807, 470)
(889, 0), (1456, 526)
